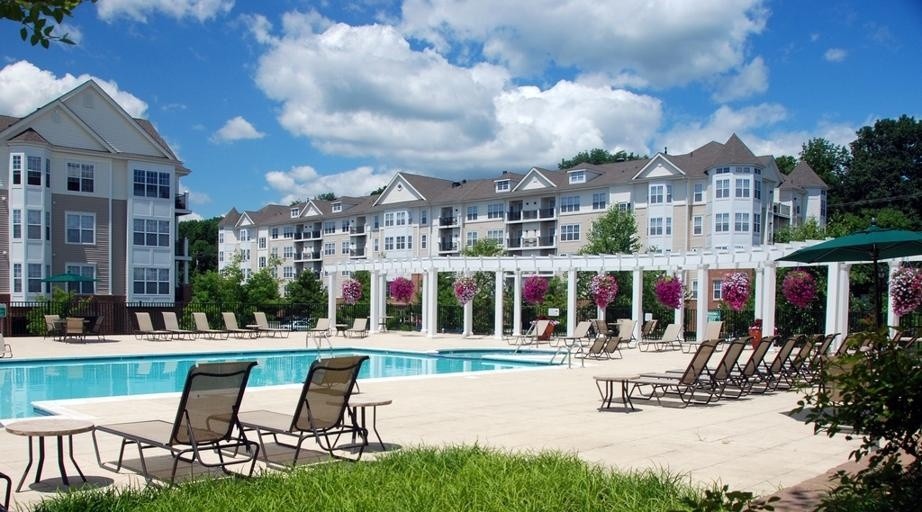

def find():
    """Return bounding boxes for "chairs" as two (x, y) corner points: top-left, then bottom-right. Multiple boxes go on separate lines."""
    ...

(220, 311), (258, 339)
(63, 316), (85, 343)
(311, 319), (333, 337)
(301, 353), (362, 394)
(347, 318), (368, 339)
(134, 311), (171, 342)
(252, 311), (291, 339)
(207, 354), (369, 474)
(161, 311), (198, 341)
(85, 315), (105, 341)
(0, 331), (14, 358)
(191, 312), (231, 340)
(43, 313), (62, 342)
(305, 317), (333, 350)
(93, 361), (261, 490)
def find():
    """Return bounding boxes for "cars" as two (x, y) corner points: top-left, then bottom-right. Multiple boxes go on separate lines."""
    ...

(281, 321), (311, 330)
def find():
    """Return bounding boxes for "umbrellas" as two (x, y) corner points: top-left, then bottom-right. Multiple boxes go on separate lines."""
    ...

(40, 270), (103, 311)
(771, 214), (921, 361)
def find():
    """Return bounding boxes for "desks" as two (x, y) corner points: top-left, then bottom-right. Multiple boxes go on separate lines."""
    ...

(53, 319), (91, 342)
(332, 324), (349, 338)
(326, 395), (393, 453)
(245, 324), (263, 339)
(3, 417), (95, 493)
